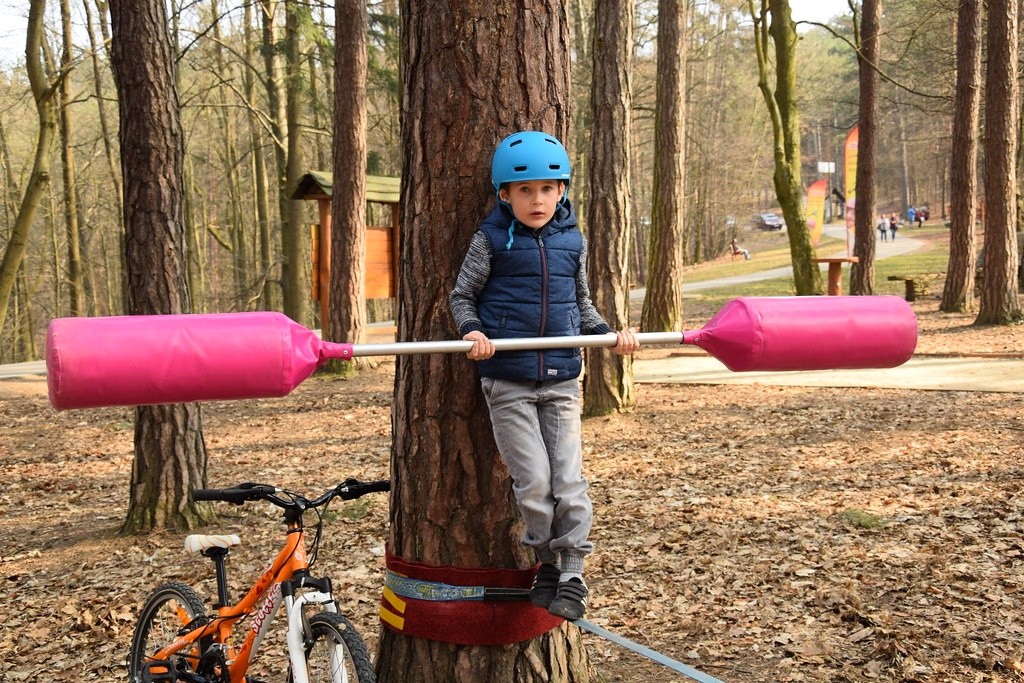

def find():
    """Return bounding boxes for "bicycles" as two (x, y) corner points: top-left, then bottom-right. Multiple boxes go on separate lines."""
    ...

(125, 476), (391, 683)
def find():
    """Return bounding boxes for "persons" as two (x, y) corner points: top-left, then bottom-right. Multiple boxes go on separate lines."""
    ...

(878, 213), (888, 242)
(889, 213), (899, 240)
(448, 131), (641, 621)
(732, 238), (750, 260)
(916, 208), (926, 228)
(907, 206), (916, 228)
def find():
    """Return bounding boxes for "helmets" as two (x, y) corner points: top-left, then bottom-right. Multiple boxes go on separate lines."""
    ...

(492, 131), (572, 193)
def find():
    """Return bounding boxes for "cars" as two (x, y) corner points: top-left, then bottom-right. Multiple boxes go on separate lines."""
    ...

(751, 214), (783, 232)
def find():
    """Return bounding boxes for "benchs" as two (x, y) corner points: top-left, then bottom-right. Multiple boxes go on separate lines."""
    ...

(730, 243), (743, 261)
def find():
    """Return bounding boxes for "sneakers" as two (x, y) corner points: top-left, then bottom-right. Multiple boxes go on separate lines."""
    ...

(547, 577), (589, 620)
(530, 563), (562, 608)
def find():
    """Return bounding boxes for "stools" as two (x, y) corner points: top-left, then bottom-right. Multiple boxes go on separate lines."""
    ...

(887, 274), (926, 302)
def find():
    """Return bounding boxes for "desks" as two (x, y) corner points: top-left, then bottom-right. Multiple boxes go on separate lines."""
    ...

(809, 256), (859, 296)
